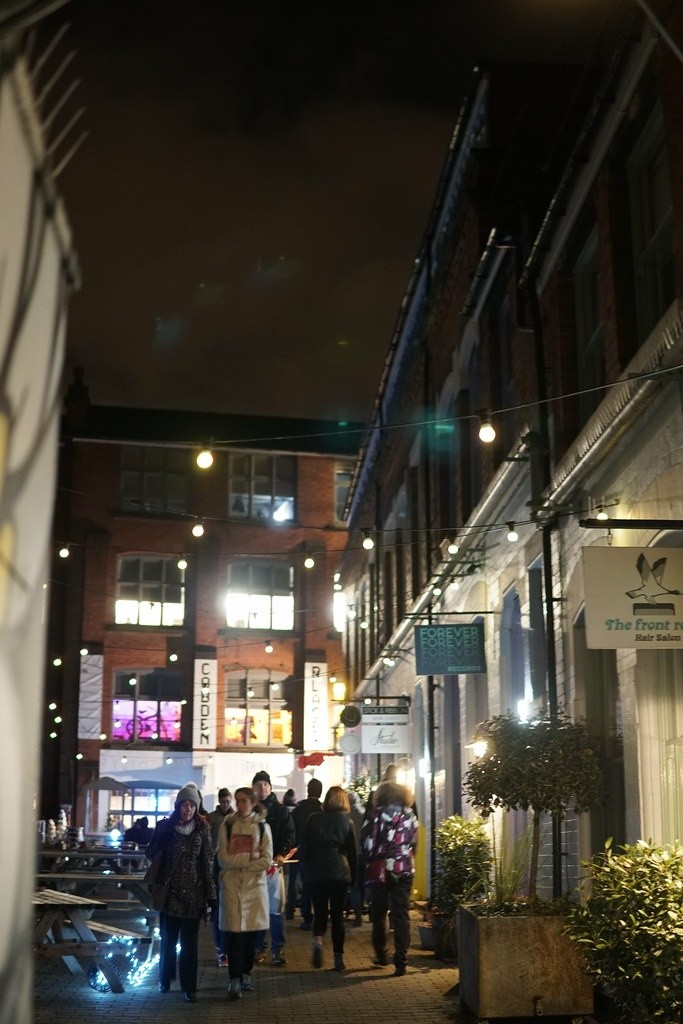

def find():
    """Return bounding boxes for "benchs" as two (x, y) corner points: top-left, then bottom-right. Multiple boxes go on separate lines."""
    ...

(34, 871), (146, 880)
(62, 919), (152, 944)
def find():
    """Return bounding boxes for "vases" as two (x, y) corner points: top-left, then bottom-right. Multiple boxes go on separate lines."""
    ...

(417, 921), (436, 949)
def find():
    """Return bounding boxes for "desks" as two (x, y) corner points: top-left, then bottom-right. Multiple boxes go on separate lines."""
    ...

(31, 846), (153, 993)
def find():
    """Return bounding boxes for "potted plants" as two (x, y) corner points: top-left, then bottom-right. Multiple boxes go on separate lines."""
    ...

(455, 701), (608, 1019)
(426, 814), (499, 960)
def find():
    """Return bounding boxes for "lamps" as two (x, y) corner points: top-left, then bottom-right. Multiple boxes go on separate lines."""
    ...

(463, 718), (495, 758)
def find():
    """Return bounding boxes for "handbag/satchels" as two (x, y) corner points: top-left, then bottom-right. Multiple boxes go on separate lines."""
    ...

(150, 865), (172, 912)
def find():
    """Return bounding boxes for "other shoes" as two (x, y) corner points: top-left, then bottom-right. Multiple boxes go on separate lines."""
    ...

(255, 950), (267, 963)
(285, 913), (294, 920)
(332, 953), (346, 971)
(373, 956), (391, 968)
(300, 922), (311, 932)
(313, 936), (322, 969)
(353, 920), (362, 927)
(159, 981), (170, 994)
(239, 973), (254, 991)
(184, 991), (196, 1003)
(271, 954), (289, 966)
(217, 955), (229, 968)
(226, 978), (244, 1000)
(394, 967), (406, 978)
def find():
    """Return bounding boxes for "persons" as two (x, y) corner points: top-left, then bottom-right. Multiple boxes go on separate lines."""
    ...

(252, 770), (295, 967)
(361, 780), (420, 976)
(300, 882), (314, 931)
(302, 786), (358, 971)
(207, 788), (236, 921)
(145, 781), (217, 1000)
(281, 788), (301, 908)
(123, 817), (155, 866)
(217, 788), (272, 1000)
(364, 763), (418, 852)
(284, 778), (323, 918)
(348, 793), (371, 927)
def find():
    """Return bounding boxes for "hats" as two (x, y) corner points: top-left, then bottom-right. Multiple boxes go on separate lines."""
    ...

(282, 789), (297, 805)
(173, 781), (201, 813)
(136, 816), (148, 829)
(252, 771), (271, 785)
